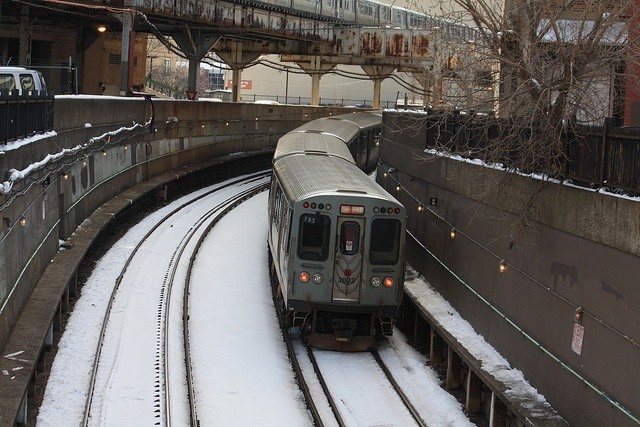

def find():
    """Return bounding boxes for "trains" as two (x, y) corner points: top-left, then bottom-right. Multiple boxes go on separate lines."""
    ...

(267, 109), (407, 351)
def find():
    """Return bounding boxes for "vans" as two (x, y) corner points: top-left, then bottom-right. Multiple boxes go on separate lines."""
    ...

(1, 66), (48, 95)
